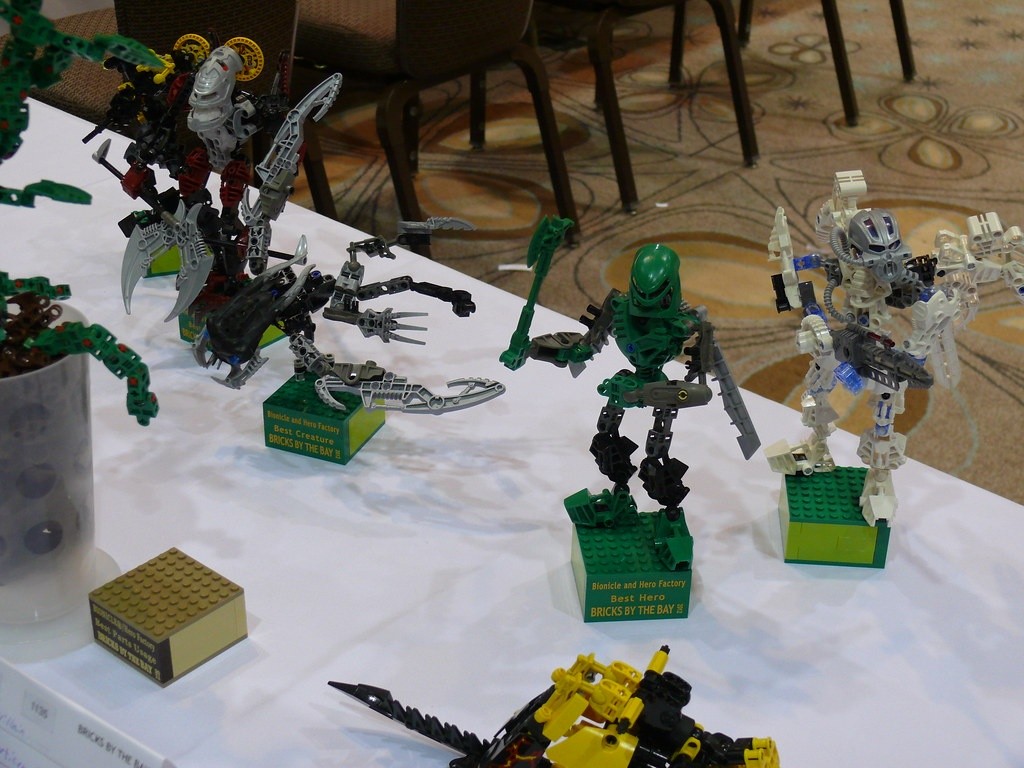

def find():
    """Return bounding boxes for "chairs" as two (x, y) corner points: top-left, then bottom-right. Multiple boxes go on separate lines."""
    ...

(0, 0), (916, 256)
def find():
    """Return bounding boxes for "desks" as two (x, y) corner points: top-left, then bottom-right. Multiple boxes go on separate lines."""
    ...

(0, 91), (1024, 767)
